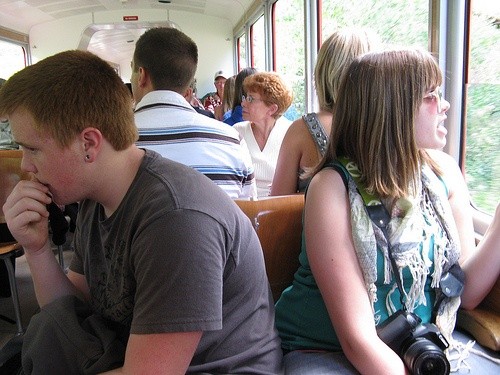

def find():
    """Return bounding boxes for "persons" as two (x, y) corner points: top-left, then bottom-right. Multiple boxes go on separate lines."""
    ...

(274, 42), (499, 375)
(0, 48), (282, 375)
(272, 28), (377, 198)
(128, 26), (258, 203)
(0, 27), (295, 299)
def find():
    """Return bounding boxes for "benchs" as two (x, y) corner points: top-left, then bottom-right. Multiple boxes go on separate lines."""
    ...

(236, 193), (500, 350)
(0, 150), (65, 336)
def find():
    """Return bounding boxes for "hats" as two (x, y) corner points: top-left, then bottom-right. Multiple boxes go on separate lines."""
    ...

(214, 71), (228, 81)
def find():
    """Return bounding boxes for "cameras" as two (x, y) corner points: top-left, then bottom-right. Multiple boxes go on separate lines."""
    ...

(379, 307), (451, 375)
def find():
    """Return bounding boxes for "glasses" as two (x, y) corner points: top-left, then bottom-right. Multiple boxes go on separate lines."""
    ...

(421, 85), (442, 114)
(242, 95), (265, 103)
